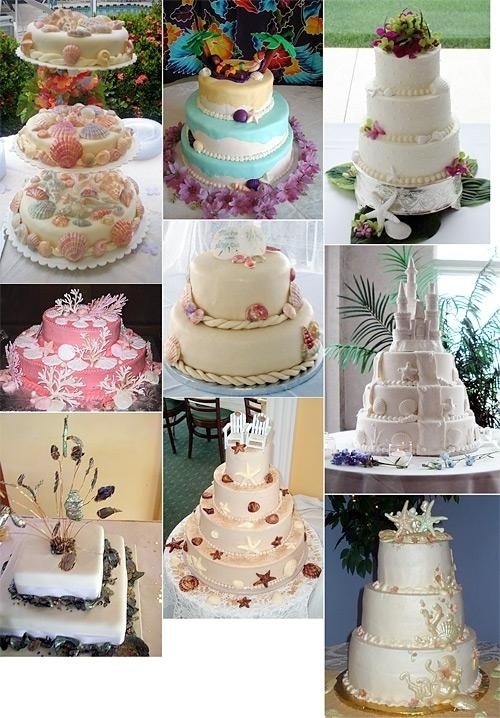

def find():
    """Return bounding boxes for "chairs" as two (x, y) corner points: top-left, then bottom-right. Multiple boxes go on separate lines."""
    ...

(245, 397), (260, 424)
(183, 397), (234, 464)
(222, 412), (249, 449)
(246, 414), (272, 450)
(164, 397), (183, 454)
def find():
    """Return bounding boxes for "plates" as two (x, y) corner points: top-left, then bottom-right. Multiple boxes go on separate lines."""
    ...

(333, 668), (490, 718)
(11, 140), (141, 175)
(164, 351), (326, 394)
(4, 200), (151, 271)
(15, 44), (137, 72)
(354, 166), (463, 217)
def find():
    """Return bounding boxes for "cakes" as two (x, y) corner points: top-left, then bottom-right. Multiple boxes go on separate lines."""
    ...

(355, 256), (480, 456)
(0, 520), (144, 659)
(164, 250), (319, 386)
(348, 10), (466, 211)
(341, 499), (488, 715)
(176, 24), (295, 190)
(10, 168), (145, 262)
(20, 7), (133, 65)
(166, 441), (323, 621)
(16, 102), (134, 168)
(3, 288), (156, 416)
